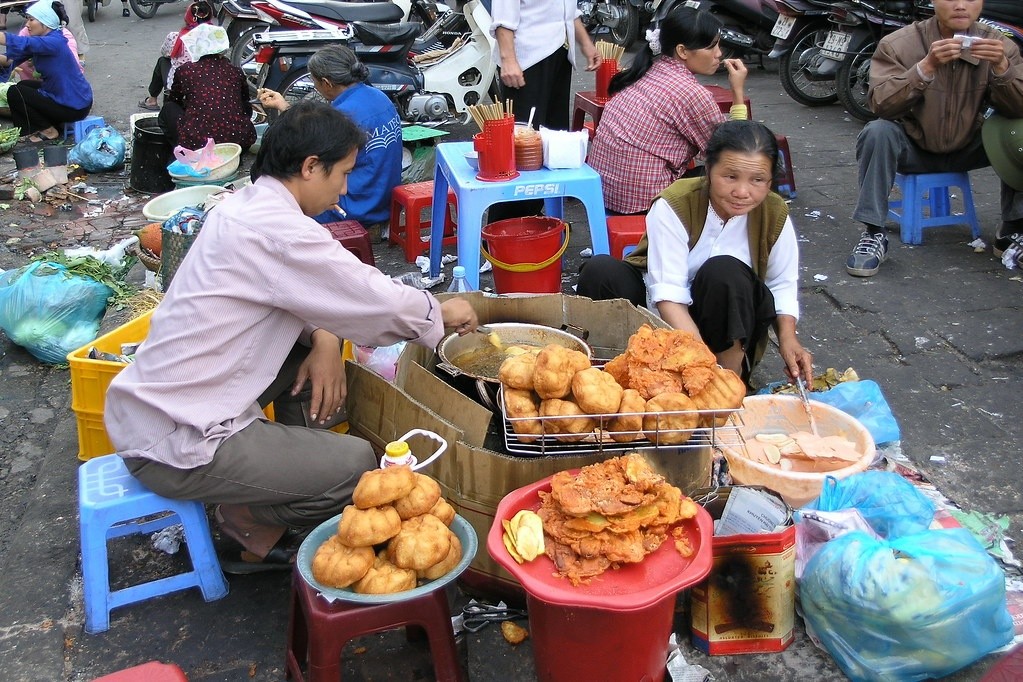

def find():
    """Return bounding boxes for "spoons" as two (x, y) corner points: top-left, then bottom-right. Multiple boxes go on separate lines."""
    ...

(528, 106), (536, 127)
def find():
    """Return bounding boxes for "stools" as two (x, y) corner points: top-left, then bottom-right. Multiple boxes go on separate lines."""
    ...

(366, 220), (390, 244)
(282, 553), (462, 682)
(387, 178), (459, 265)
(428, 139), (610, 291)
(63, 115), (105, 144)
(569, 91), (609, 164)
(771, 133), (798, 199)
(317, 219), (375, 268)
(606, 214), (646, 262)
(703, 85), (752, 120)
(77, 453), (231, 636)
(887, 172), (981, 246)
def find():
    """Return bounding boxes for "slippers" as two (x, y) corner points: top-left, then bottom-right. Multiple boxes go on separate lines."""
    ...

(217, 524), (317, 574)
(137, 96), (160, 110)
(24, 131), (54, 146)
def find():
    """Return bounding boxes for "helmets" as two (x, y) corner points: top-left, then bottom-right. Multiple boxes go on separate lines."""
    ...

(981, 113), (1023, 195)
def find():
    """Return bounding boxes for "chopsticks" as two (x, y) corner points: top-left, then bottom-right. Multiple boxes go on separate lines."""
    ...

(595, 38), (626, 65)
(466, 96), (513, 129)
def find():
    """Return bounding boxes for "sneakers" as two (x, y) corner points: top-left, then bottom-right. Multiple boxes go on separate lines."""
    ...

(845, 231), (892, 277)
(992, 222), (1023, 269)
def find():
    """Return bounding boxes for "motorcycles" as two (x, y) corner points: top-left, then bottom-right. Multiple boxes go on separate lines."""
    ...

(1, 0), (1023, 154)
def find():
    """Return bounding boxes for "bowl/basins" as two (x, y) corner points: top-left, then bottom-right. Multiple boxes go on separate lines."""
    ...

(166, 141), (240, 180)
(142, 183), (227, 220)
(706, 392), (875, 505)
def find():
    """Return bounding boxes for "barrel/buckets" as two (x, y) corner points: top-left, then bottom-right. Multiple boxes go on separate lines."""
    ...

(523, 582), (677, 680)
(129, 117), (178, 195)
(480, 216), (570, 294)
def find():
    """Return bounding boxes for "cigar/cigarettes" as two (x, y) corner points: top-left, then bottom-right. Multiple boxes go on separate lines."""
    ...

(333, 202), (346, 217)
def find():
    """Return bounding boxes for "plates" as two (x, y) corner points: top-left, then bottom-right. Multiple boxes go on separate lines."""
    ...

(298, 505), (480, 602)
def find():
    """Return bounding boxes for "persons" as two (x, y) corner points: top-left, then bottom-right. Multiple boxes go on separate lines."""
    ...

(0, 1), (94, 146)
(489, 0), (604, 132)
(120, 0), (130, 17)
(844, 0), (1023, 278)
(574, 119), (816, 393)
(137, 0), (216, 107)
(158, 21), (257, 160)
(587, 5), (751, 214)
(14, 4), (85, 82)
(101, 100), (479, 569)
(56, 0), (90, 69)
(251, 42), (406, 227)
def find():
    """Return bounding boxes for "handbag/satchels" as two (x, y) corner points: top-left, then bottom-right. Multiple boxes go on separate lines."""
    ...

(793, 468), (936, 538)
(0, 253), (116, 364)
(799, 526), (1015, 682)
(68, 125), (127, 173)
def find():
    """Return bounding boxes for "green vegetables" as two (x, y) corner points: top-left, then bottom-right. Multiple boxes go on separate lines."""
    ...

(27, 251), (141, 310)
(0, 126), (21, 144)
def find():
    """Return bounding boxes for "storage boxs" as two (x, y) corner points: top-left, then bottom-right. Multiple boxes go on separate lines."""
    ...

(684, 485), (797, 657)
(65, 306), (356, 463)
(344, 291), (716, 612)
